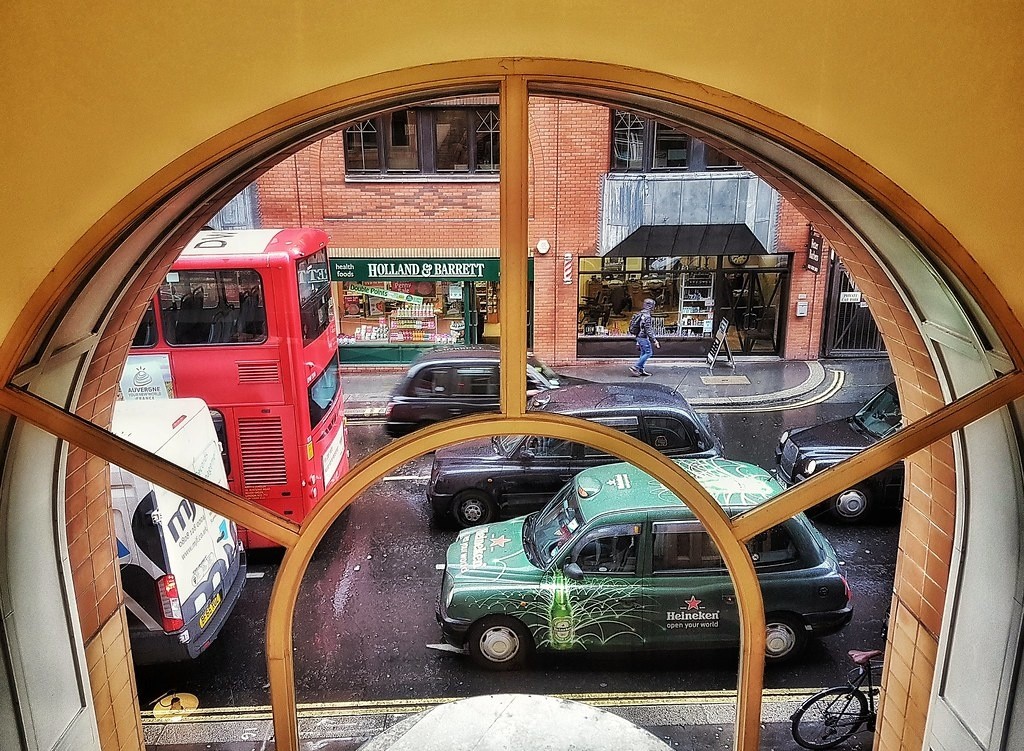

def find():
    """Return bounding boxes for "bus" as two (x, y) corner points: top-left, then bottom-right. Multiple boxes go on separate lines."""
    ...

(108, 396), (248, 689)
(113, 227), (350, 552)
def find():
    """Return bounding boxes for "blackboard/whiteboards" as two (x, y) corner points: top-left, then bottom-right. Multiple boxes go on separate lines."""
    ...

(706, 317), (735, 370)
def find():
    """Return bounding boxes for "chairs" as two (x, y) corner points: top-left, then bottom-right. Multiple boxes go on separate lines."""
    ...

(143, 284), (260, 345)
(578, 290), (612, 330)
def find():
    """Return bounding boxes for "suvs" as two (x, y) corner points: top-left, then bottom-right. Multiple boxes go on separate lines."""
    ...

(773, 381), (906, 527)
(433, 458), (854, 669)
(381, 349), (601, 456)
(426, 382), (725, 529)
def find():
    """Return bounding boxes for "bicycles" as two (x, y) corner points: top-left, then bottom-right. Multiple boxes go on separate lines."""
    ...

(789, 650), (886, 750)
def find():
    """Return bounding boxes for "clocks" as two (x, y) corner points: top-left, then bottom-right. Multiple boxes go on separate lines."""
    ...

(728, 255), (749, 266)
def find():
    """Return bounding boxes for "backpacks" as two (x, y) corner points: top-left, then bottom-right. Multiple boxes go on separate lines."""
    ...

(629, 314), (643, 335)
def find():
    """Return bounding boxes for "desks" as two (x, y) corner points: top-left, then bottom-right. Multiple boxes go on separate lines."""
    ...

(720, 304), (777, 330)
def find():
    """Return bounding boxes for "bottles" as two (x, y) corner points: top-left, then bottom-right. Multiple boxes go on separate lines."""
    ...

(488, 287), (492, 296)
(493, 305), (497, 313)
(422, 320), (429, 328)
(683, 308), (689, 313)
(398, 308), (412, 317)
(696, 478), (772, 494)
(488, 296), (492, 305)
(697, 293), (701, 300)
(413, 331), (425, 341)
(493, 295), (497, 305)
(488, 305), (492, 313)
(420, 303), (434, 317)
(403, 330), (413, 340)
(399, 319), (415, 328)
(407, 303), (420, 316)
(550, 575), (573, 651)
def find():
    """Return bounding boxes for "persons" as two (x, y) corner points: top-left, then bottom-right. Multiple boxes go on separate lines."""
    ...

(628, 298), (661, 376)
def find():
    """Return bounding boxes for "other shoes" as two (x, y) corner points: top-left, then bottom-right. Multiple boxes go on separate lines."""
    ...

(642, 370), (653, 376)
(629, 366), (641, 377)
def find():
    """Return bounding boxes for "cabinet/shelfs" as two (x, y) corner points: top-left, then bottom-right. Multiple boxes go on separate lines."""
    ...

(388, 315), (438, 344)
(461, 281), (488, 313)
(678, 272), (714, 337)
